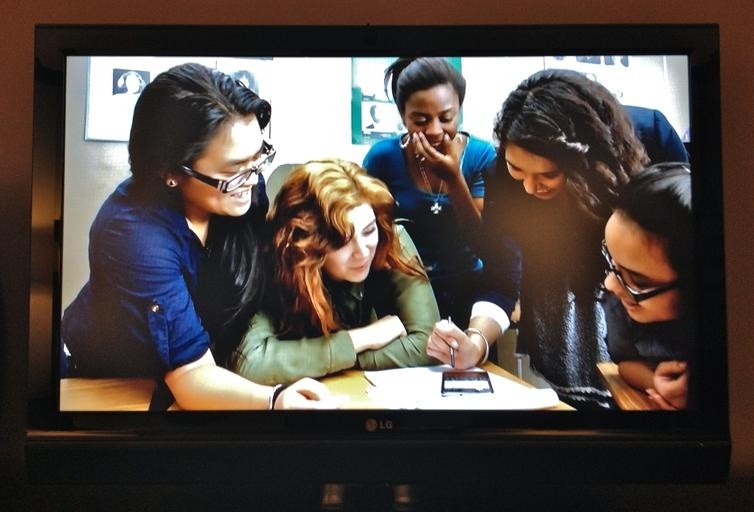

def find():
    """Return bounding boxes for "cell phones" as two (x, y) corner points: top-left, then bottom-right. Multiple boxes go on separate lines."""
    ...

(441, 370), (493, 396)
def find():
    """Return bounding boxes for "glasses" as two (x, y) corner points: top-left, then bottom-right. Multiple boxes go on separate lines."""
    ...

(180, 140), (277, 193)
(601, 239), (680, 305)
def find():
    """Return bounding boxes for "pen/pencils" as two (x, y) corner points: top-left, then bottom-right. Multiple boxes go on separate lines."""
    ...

(448, 315), (455, 367)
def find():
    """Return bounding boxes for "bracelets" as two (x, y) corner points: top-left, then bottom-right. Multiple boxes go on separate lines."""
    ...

(270, 383), (287, 411)
(463, 328), (489, 365)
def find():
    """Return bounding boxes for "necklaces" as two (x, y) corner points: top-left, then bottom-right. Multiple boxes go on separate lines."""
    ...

(418, 164), (446, 215)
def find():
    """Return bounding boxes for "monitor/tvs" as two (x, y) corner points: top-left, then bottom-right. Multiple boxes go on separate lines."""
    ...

(24, 20), (733, 510)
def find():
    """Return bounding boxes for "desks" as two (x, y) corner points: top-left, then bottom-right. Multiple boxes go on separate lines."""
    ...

(55, 336), (578, 412)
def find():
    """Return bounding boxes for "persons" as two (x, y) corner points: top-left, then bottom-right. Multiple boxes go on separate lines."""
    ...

(427, 69), (689, 407)
(361, 57), (500, 364)
(600, 162), (692, 409)
(237, 159), (440, 386)
(61, 64), (330, 414)
